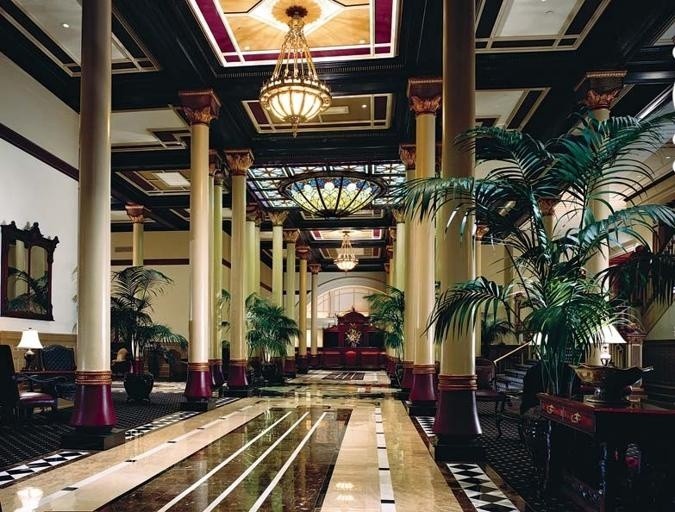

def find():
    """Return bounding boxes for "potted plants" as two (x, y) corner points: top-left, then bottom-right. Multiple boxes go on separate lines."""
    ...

(256, 302), (302, 381)
(241, 289), (275, 386)
(65, 258), (189, 409)
(381, 101), (674, 478)
(362, 278), (444, 385)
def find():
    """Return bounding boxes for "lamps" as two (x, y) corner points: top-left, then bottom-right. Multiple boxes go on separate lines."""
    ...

(256, 0), (333, 142)
(12, 326), (46, 374)
(331, 230), (360, 277)
(584, 320), (629, 368)
(275, 166), (391, 226)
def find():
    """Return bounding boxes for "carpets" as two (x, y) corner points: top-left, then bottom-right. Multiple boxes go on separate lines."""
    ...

(474, 401), (641, 512)
(0, 390), (190, 473)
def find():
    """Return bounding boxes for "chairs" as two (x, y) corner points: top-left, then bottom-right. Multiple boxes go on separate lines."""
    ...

(0, 336), (186, 435)
(516, 356), (597, 445)
(475, 354), (510, 440)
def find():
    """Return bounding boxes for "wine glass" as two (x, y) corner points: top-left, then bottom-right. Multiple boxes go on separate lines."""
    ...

(568, 362), (657, 407)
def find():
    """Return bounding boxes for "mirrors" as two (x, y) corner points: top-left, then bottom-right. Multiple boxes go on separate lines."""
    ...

(0, 220), (60, 322)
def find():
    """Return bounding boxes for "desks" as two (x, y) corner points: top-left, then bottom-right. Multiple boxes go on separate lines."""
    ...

(532, 386), (675, 512)
